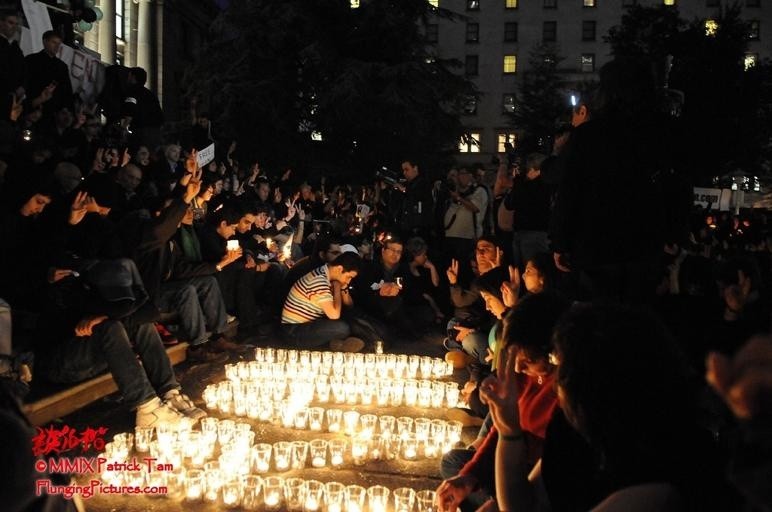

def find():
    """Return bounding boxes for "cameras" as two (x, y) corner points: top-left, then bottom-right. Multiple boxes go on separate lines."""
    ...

(440, 175), (456, 192)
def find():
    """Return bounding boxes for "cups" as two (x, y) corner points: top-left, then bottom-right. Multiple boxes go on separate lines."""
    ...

(98, 346), (462, 512)
(374, 341), (385, 353)
(225, 239), (241, 251)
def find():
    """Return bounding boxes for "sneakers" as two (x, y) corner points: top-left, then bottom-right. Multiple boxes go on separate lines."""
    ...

(442, 337), (451, 351)
(132, 393), (197, 434)
(161, 387), (209, 421)
(329, 336), (366, 352)
(207, 336), (248, 355)
(185, 344), (230, 366)
(443, 350), (473, 369)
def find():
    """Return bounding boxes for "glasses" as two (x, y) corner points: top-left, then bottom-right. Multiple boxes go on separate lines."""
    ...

(386, 247), (403, 252)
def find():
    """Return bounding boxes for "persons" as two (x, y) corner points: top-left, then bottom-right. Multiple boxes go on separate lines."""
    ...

(0, 0), (771, 510)
